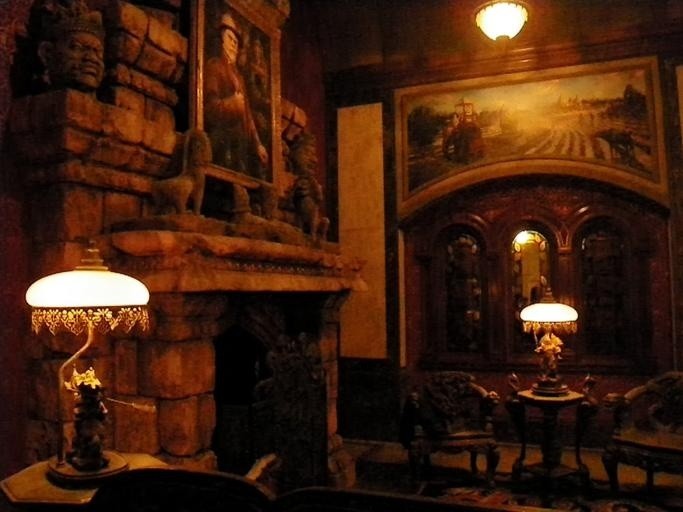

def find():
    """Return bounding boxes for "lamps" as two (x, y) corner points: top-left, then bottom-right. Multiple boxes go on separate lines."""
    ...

(470, 1), (531, 54)
(25, 241), (151, 489)
(520, 301), (579, 397)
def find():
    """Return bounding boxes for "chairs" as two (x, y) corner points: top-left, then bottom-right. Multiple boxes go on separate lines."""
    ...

(600, 371), (683, 506)
(402, 371), (501, 496)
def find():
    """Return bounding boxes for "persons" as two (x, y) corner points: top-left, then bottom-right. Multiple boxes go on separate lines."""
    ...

(34, 11), (109, 98)
(204, 13), (266, 175)
(288, 131), (319, 178)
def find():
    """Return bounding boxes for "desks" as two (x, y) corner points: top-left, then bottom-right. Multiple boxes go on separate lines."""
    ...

(504, 373), (598, 494)
(0, 451), (170, 512)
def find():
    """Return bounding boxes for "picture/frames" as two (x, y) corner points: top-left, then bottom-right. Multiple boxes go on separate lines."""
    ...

(188, 1), (291, 197)
